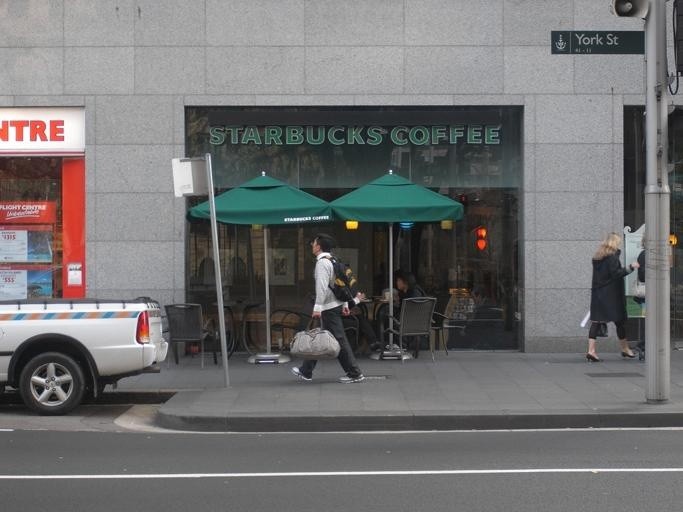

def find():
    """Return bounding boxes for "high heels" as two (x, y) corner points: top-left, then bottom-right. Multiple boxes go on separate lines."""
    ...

(586, 354), (603, 363)
(621, 351), (638, 358)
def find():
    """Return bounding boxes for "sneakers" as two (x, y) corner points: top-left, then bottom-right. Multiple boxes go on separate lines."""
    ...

(290, 366), (312, 382)
(337, 373), (366, 384)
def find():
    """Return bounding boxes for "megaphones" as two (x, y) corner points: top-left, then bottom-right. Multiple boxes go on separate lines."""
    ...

(609, 0), (650, 18)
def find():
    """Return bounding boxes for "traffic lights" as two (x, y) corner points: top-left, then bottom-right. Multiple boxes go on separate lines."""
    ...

(468, 226), (489, 258)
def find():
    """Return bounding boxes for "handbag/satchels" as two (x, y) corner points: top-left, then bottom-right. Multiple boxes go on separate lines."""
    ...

(595, 322), (607, 337)
(633, 275), (644, 299)
(290, 316), (340, 361)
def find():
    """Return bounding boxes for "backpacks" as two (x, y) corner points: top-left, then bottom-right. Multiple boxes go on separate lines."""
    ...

(320, 255), (358, 303)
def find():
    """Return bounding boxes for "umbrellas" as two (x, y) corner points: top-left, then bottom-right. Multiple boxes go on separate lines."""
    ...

(325, 168), (464, 346)
(184, 170), (332, 354)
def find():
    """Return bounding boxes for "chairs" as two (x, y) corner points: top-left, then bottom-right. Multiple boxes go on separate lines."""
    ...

(312, 293), (451, 366)
(164, 288), (318, 370)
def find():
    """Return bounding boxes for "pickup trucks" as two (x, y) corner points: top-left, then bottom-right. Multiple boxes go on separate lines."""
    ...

(0, 297), (169, 414)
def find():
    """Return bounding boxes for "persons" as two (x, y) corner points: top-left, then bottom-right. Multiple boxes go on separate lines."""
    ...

(585, 231), (640, 362)
(631, 232), (645, 361)
(290, 232), (365, 384)
(340, 291), (389, 353)
(376, 271), (427, 359)
